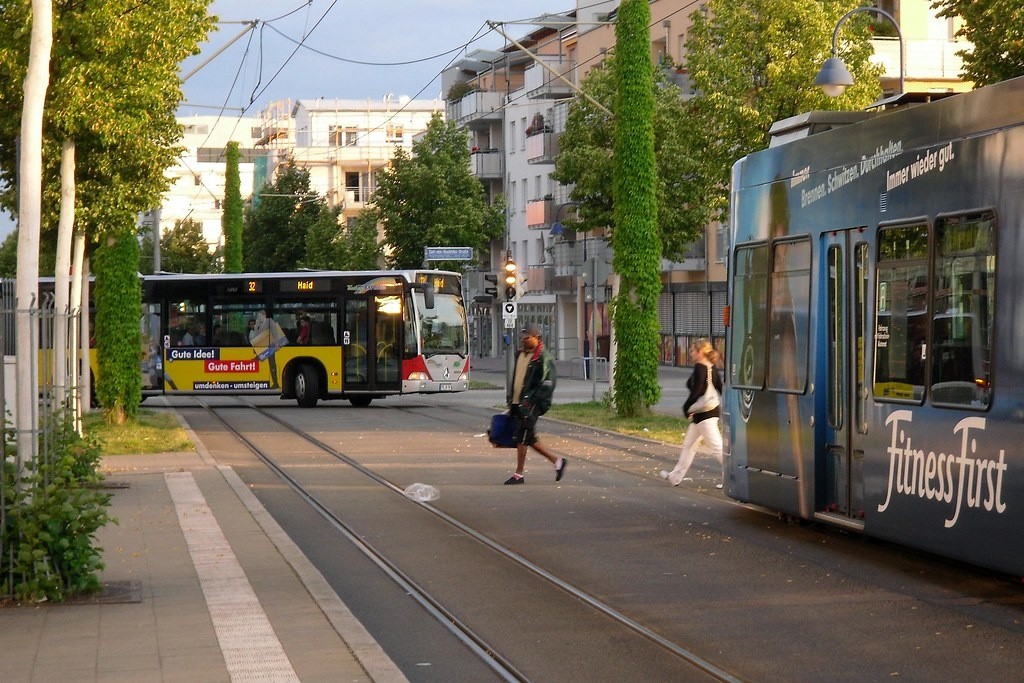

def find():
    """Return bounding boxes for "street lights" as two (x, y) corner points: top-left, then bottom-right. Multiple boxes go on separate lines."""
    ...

(549, 203), (590, 379)
(814, 8), (908, 97)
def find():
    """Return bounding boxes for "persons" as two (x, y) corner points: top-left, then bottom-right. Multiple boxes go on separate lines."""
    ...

(181, 322), (223, 347)
(743, 177), (807, 517)
(660, 338), (724, 488)
(242, 319), (256, 345)
(503, 324), (567, 484)
(296, 316), (311, 344)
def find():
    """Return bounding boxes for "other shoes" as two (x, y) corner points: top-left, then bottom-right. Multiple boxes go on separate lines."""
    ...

(659, 470), (679, 487)
(555, 457), (567, 482)
(505, 477), (524, 484)
(716, 484), (722, 488)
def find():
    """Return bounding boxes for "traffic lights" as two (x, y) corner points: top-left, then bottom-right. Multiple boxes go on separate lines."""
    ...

(484, 273), (501, 298)
(505, 260), (517, 299)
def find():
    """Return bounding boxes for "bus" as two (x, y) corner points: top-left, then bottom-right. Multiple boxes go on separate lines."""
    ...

(0, 269), (473, 407)
(721, 80), (1024, 577)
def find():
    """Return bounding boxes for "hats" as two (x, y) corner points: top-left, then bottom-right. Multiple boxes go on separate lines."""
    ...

(519, 324), (542, 338)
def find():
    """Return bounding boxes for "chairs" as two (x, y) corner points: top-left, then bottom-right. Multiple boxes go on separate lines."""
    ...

(168, 321), (336, 347)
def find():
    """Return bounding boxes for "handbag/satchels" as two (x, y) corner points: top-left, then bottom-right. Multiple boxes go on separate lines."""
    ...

(489, 415), (518, 448)
(687, 365), (719, 413)
